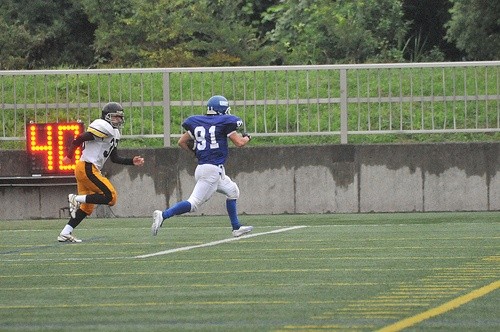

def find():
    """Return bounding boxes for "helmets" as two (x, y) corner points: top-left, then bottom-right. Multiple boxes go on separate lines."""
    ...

(102, 102), (125, 128)
(207, 96), (229, 116)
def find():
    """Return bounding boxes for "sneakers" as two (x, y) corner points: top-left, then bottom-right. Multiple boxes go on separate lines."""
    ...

(232, 226), (253, 237)
(152, 210), (163, 236)
(68, 194), (81, 219)
(57, 233), (82, 244)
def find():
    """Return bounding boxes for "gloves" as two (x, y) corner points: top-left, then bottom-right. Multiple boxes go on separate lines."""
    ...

(243, 133), (252, 141)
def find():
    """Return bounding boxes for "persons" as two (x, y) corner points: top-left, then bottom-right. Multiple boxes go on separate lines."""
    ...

(55, 101), (145, 242)
(151, 94), (254, 238)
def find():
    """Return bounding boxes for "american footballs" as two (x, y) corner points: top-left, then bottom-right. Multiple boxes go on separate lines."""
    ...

(186, 140), (194, 152)
